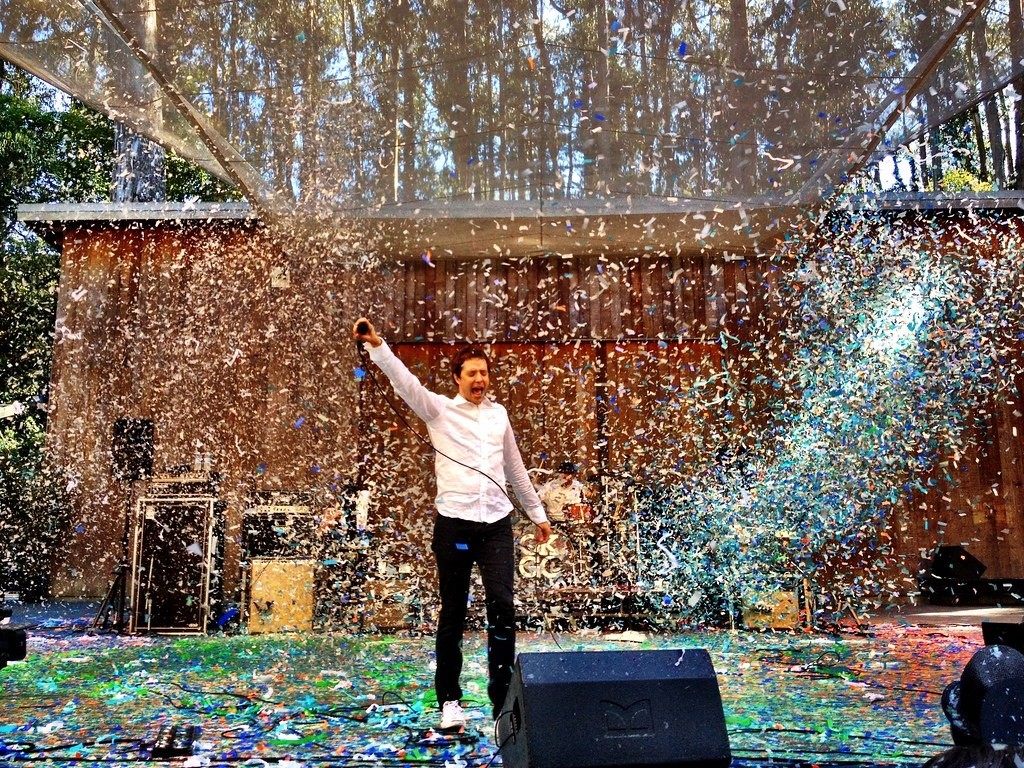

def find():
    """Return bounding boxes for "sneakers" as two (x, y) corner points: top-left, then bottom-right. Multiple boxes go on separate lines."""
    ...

(440, 701), (467, 730)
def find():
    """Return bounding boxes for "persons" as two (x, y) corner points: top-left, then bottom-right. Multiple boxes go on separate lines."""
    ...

(536, 462), (600, 527)
(353, 317), (551, 730)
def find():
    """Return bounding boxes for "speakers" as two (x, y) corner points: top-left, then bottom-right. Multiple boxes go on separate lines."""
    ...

(931, 545), (987, 581)
(498, 648), (732, 768)
(111, 418), (154, 480)
(243, 514), (314, 560)
(251, 560), (315, 636)
(741, 589), (799, 630)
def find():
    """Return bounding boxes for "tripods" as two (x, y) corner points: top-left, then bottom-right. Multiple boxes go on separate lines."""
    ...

(89, 482), (135, 636)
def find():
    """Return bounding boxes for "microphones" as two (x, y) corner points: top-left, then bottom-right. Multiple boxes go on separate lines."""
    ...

(357, 322), (369, 352)
